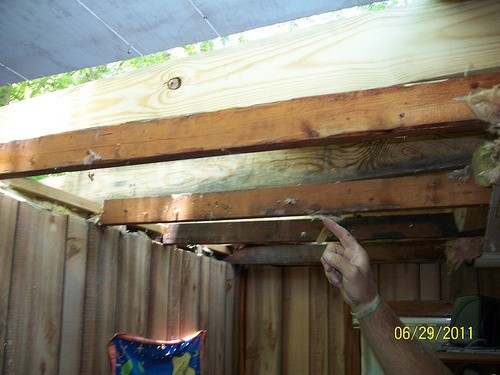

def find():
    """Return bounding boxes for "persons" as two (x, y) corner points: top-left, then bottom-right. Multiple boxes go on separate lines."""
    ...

(319, 216), (457, 375)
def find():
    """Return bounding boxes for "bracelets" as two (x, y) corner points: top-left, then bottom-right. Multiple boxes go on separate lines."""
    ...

(350, 291), (382, 320)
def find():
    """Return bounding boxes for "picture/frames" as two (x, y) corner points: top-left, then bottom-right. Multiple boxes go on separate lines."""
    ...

(343, 301), (453, 375)
